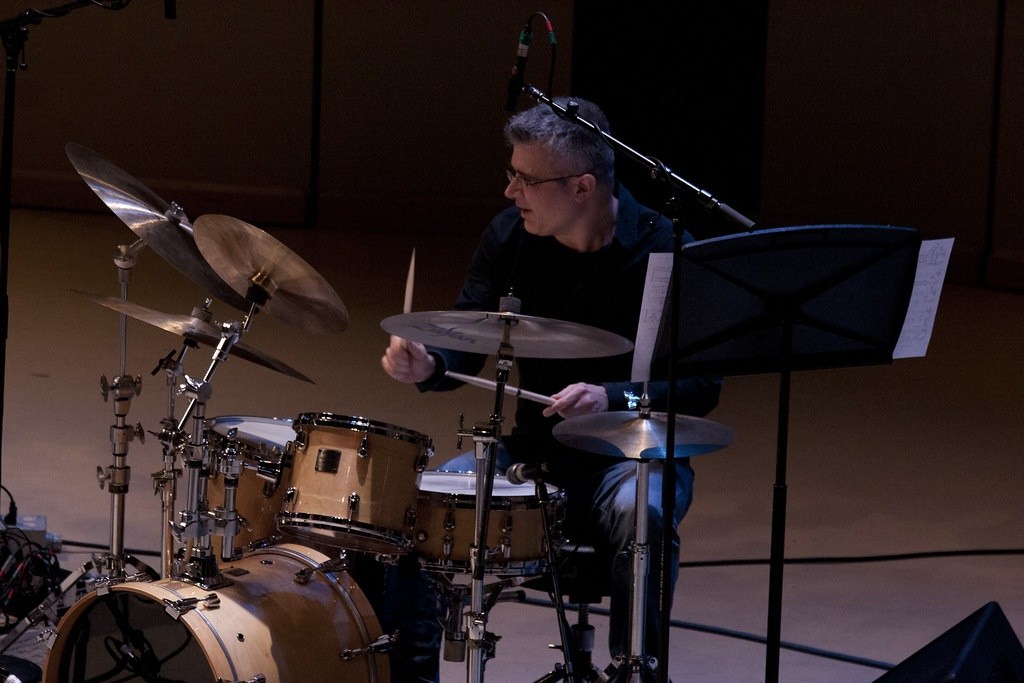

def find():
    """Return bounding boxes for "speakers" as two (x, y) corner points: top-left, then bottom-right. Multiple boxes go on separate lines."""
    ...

(873, 602), (1024, 683)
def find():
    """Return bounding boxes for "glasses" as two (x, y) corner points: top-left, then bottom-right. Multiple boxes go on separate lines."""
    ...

(506, 167), (588, 190)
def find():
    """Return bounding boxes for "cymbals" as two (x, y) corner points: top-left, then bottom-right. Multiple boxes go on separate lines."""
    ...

(549, 410), (736, 460)
(191, 212), (352, 336)
(68, 289), (319, 386)
(64, 142), (261, 317)
(377, 309), (636, 360)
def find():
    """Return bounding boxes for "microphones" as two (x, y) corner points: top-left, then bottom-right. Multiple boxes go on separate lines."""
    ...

(506, 22), (534, 113)
(114, 638), (157, 683)
(506, 463), (551, 485)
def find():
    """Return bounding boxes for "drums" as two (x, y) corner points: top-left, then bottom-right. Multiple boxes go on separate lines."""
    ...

(202, 413), (299, 564)
(275, 410), (436, 563)
(409, 470), (569, 573)
(33, 543), (399, 683)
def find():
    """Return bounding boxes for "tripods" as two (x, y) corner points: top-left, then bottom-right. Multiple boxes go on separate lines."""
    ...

(597, 457), (671, 683)
(0, 238), (161, 654)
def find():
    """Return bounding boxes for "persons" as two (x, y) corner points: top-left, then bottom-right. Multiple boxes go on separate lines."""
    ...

(382, 97), (723, 683)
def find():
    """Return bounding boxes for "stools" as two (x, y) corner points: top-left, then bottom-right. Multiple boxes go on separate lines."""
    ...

(534, 542), (609, 683)
